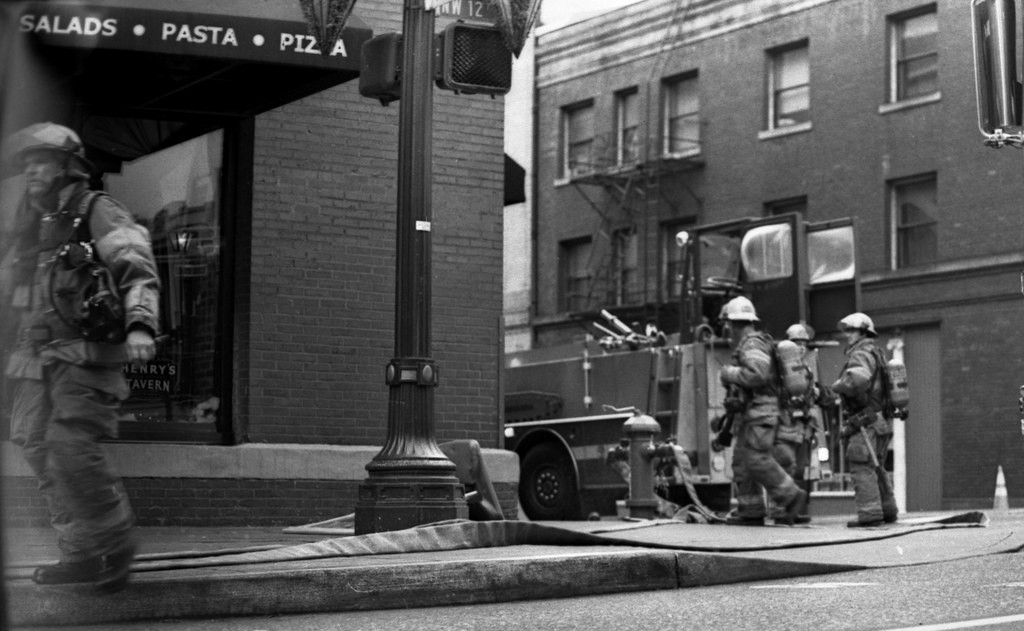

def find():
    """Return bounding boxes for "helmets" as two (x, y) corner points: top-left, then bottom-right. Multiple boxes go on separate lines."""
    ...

(787, 324), (811, 340)
(12, 122), (95, 170)
(840, 313), (878, 337)
(719, 295), (760, 321)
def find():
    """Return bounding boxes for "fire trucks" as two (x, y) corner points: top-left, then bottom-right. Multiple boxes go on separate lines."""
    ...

(502, 211), (865, 523)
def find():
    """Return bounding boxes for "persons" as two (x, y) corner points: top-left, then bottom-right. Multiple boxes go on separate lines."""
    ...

(718, 296), (820, 526)
(831, 312), (899, 528)
(0, 121), (160, 588)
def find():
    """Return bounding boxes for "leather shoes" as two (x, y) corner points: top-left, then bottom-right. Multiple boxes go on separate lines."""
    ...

(33, 558), (87, 584)
(90, 548), (128, 594)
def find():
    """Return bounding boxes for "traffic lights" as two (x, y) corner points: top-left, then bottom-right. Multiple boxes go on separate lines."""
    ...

(436, 19), (512, 96)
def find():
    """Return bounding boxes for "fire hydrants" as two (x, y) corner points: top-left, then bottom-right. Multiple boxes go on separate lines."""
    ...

(617, 411), (670, 519)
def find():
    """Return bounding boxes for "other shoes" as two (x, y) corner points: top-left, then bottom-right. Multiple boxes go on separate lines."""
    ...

(882, 515), (898, 524)
(727, 515), (764, 526)
(847, 519), (881, 527)
(774, 515), (811, 527)
(783, 488), (807, 524)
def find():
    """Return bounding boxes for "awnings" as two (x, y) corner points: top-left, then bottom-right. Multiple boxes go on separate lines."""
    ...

(0, 0), (373, 116)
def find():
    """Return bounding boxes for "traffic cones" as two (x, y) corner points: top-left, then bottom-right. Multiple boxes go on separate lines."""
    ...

(992, 465), (1011, 511)
